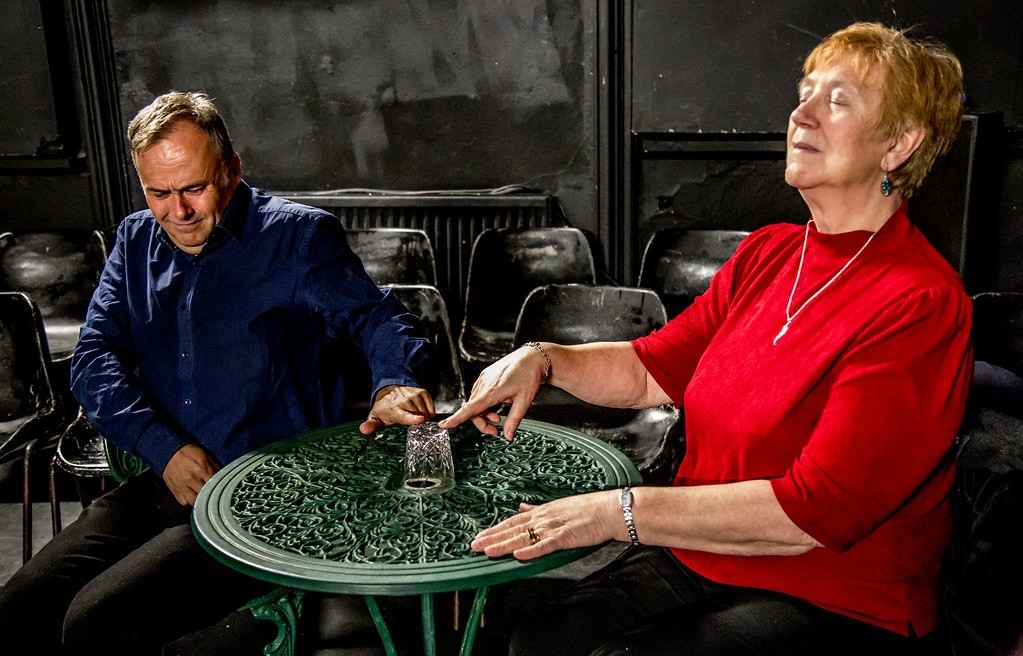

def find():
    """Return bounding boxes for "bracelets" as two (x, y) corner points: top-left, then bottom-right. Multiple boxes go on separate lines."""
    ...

(523, 341), (552, 384)
(368, 417), (380, 428)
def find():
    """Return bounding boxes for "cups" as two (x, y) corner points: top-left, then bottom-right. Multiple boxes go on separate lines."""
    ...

(403, 422), (455, 494)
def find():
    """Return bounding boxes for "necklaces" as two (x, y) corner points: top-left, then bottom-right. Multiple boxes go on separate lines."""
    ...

(774, 220), (878, 345)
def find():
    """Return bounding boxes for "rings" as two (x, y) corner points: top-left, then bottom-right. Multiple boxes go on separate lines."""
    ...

(526, 527), (541, 546)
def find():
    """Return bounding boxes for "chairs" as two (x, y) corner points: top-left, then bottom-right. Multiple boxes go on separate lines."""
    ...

(0, 219), (1023, 656)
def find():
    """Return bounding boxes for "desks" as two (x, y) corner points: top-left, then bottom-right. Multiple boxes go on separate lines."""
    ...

(192, 412), (645, 656)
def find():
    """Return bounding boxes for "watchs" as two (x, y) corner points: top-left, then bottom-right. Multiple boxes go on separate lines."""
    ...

(622, 485), (640, 546)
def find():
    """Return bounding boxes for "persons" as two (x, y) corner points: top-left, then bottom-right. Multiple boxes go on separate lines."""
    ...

(0, 90), (437, 656)
(438, 22), (974, 656)
